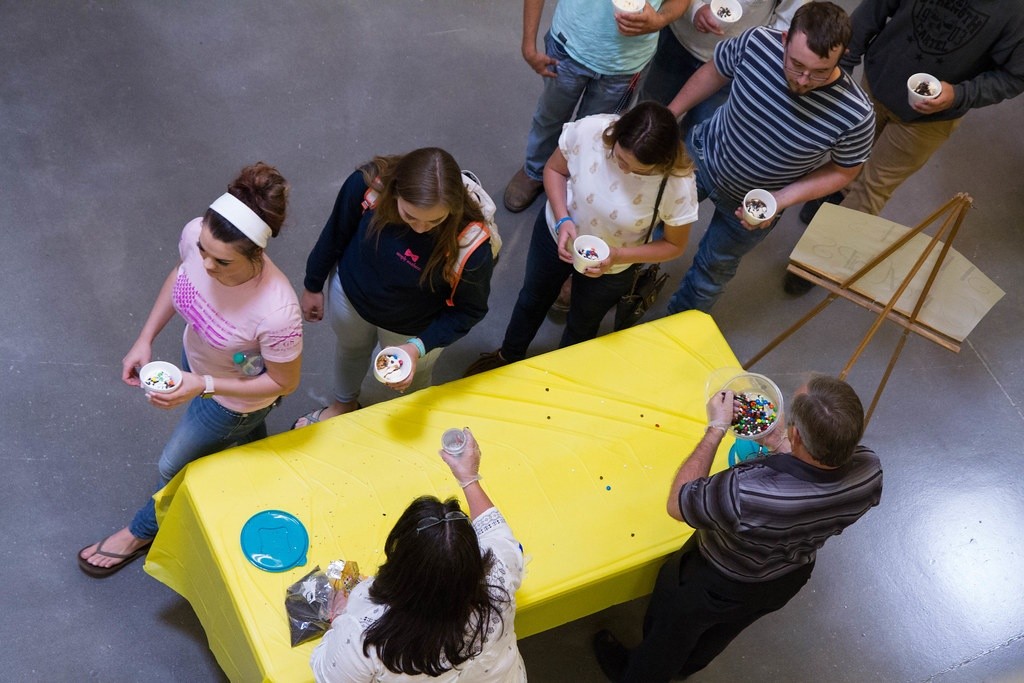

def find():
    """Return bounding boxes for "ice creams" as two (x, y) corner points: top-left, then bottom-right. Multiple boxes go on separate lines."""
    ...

(911, 81), (935, 96)
(613, 0), (640, 11)
(377, 354), (404, 381)
(144, 368), (174, 389)
(715, 5), (737, 20)
(745, 198), (768, 220)
(578, 247), (599, 261)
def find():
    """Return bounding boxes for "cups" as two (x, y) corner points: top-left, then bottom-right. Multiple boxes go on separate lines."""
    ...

(373, 346), (412, 384)
(139, 360), (183, 398)
(907, 72), (942, 110)
(710, 0), (743, 29)
(611, 0), (645, 15)
(741, 188), (776, 225)
(440, 428), (467, 458)
(571, 234), (611, 274)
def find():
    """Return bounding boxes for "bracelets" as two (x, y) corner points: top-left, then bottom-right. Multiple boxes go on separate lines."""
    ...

(405, 338), (426, 358)
(555, 216), (573, 235)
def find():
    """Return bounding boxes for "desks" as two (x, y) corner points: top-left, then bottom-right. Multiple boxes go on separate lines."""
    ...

(142, 308), (752, 683)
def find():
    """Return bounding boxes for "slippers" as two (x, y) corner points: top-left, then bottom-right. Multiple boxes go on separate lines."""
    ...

(291, 401), (362, 431)
(77, 530), (153, 577)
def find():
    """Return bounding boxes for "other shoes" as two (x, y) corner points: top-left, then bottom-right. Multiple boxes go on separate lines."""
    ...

(463, 347), (510, 377)
(550, 275), (573, 313)
(785, 269), (814, 295)
(592, 630), (632, 683)
(799, 192), (846, 224)
(504, 166), (543, 214)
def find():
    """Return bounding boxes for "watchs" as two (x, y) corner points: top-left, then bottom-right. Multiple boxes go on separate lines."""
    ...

(201, 374), (215, 401)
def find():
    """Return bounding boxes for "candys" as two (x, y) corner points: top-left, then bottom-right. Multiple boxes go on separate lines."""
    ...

(729, 395), (778, 436)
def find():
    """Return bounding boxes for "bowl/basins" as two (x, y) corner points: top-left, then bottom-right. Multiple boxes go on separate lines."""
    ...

(718, 373), (785, 440)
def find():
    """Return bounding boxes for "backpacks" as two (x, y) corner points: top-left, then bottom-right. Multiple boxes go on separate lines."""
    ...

(361, 164), (503, 309)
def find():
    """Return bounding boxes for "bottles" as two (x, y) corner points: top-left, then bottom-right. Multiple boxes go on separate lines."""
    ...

(233, 352), (267, 376)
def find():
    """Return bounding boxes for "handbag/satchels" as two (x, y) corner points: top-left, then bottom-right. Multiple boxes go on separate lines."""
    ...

(622, 262), (669, 327)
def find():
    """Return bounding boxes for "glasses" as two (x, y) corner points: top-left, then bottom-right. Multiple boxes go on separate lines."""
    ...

(783, 40), (838, 83)
(411, 511), (470, 543)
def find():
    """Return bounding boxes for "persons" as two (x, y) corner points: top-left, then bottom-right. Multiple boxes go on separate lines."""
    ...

(591, 376), (882, 682)
(504, 1), (691, 214)
(308, 426), (528, 683)
(630, 0), (779, 140)
(290, 146), (501, 433)
(784, 2), (1024, 298)
(465, 98), (699, 383)
(550, 1), (876, 315)
(77, 160), (303, 578)
